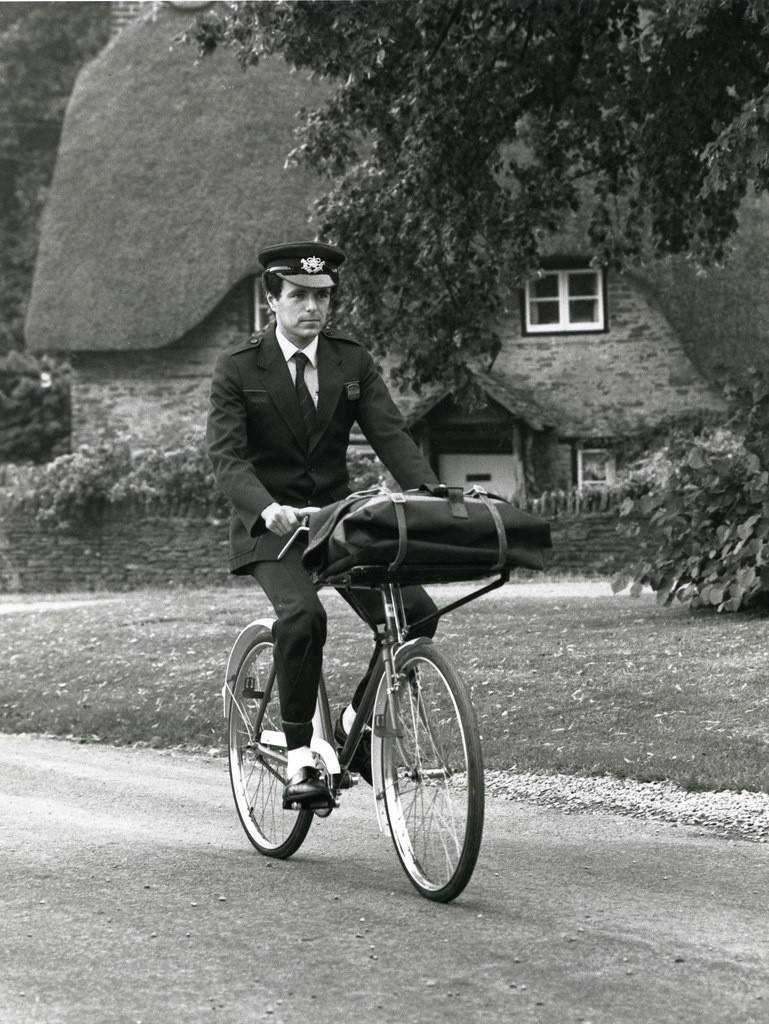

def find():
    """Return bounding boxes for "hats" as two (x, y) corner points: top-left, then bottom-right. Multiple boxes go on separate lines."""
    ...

(256, 241), (346, 288)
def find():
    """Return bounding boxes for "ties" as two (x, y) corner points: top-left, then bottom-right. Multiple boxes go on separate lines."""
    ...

(291, 353), (316, 441)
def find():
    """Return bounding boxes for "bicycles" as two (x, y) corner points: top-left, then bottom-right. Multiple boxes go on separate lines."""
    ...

(220, 506), (511, 903)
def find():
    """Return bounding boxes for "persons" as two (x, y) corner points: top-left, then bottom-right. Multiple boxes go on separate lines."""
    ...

(205, 242), (439, 810)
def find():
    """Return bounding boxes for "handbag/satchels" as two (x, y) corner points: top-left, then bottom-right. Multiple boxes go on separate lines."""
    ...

(301, 483), (552, 588)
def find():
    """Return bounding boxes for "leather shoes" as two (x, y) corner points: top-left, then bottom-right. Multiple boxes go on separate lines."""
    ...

(282, 766), (330, 801)
(334, 708), (372, 786)
